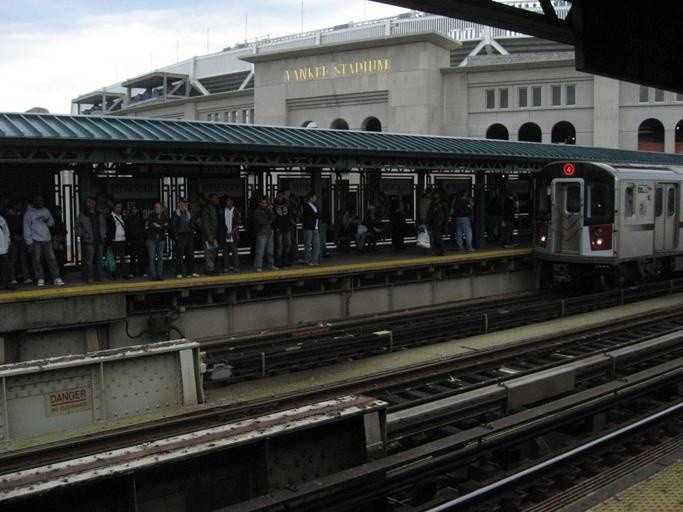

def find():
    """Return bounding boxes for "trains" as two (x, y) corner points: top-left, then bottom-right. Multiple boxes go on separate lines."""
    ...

(532, 160), (683, 289)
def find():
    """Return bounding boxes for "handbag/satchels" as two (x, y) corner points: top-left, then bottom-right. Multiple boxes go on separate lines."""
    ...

(416, 225), (431, 248)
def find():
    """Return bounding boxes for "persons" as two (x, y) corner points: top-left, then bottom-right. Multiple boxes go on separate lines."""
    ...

(343, 205), (385, 252)
(272, 189), (301, 268)
(488, 189), (521, 248)
(303, 196), (333, 258)
(104, 201), (133, 280)
(172, 198), (201, 279)
(125, 205), (149, 278)
(23, 197), (65, 287)
(199, 193), (222, 276)
(147, 203), (170, 280)
(253, 196), (280, 272)
(454, 192), (474, 252)
(220, 196), (242, 272)
(302, 192), (322, 267)
(76, 197), (105, 282)
(1, 197), (34, 285)
(427, 191), (449, 256)
(387, 205), (417, 248)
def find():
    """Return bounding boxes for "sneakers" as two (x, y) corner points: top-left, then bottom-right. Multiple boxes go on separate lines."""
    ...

(38, 279), (45, 287)
(53, 279), (64, 286)
